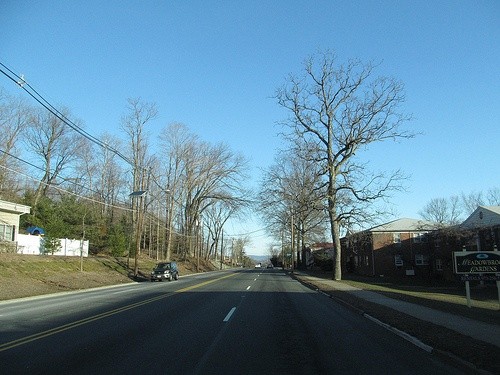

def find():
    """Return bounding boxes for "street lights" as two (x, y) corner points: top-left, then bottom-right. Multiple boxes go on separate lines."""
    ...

(128, 190), (169, 278)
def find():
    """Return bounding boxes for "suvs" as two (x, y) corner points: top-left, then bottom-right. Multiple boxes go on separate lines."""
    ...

(151, 262), (179, 281)
